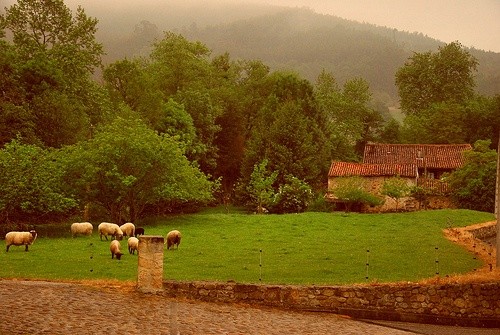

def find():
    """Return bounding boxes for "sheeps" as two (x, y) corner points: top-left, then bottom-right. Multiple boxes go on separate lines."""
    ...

(127, 236), (139, 256)
(97, 222), (124, 241)
(166, 230), (181, 250)
(110, 239), (125, 260)
(135, 227), (144, 238)
(5, 230), (39, 253)
(71, 222), (94, 238)
(119, 222), (136, 240)
